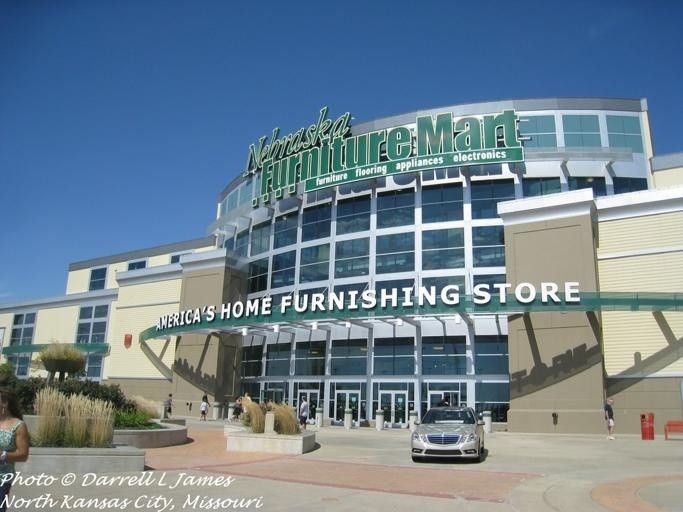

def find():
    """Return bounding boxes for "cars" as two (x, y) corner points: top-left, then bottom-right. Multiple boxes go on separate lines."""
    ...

(410, 406), (486, 463)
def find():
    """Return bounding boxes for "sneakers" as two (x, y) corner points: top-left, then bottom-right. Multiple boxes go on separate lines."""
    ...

(609, 437), (615, 440)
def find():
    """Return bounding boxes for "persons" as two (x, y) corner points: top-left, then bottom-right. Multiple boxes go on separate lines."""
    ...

(604, 399), (615, 440)
(200, 399), (207, 422)
(0, 385), (31, 512)
(202, 390), (208, 420)
(229, 392), (308, 430)
(167, 394), (172, 416)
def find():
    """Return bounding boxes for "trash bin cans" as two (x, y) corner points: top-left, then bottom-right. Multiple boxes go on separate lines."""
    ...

(640, 413), (654, 440)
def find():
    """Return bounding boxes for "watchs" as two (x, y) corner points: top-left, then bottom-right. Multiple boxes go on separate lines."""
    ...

(0, 451), (7, 461)
